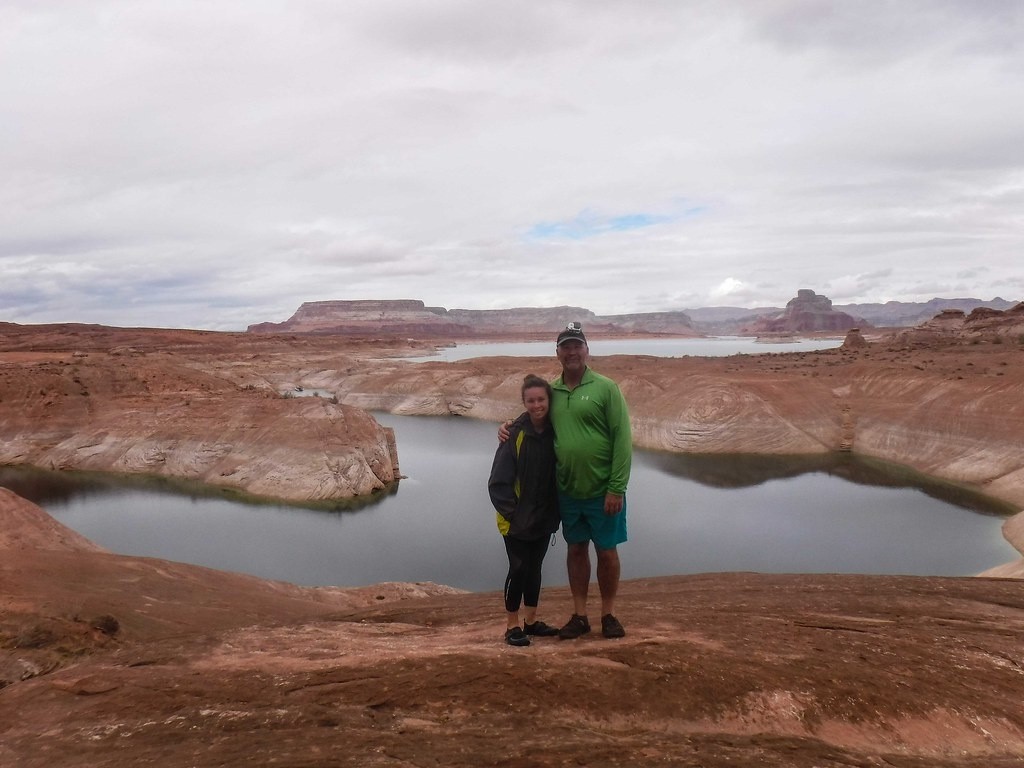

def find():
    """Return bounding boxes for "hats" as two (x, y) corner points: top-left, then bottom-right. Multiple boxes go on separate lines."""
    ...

(557, 327), (587, 347)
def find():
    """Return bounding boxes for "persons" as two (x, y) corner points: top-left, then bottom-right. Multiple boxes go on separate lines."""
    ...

(488, 374), (561, 646)
(498, 323), (632, 639)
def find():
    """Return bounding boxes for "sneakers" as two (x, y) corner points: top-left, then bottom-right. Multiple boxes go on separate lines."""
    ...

(558, 613), (591, 640)
(522, 618), (559, 637)
(505, 627), (530, 646)
(601, 614), (625, 639)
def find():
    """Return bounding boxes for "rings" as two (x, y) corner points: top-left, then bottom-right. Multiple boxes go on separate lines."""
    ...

(498, 435), (500, 438)
